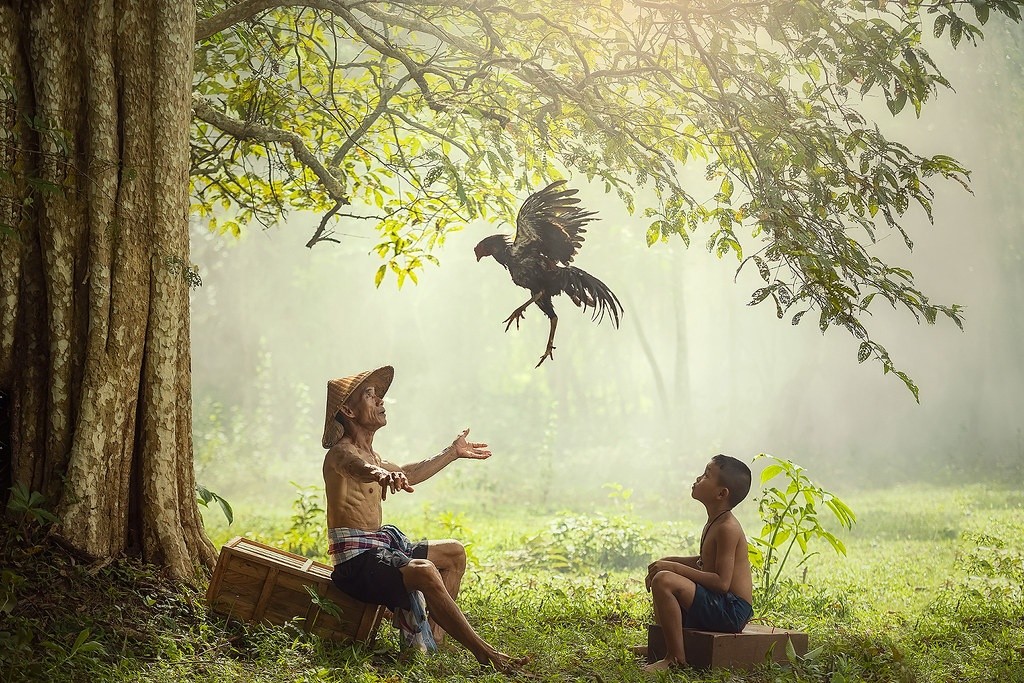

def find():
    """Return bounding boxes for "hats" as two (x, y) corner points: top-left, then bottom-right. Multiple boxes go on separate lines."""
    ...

(322, 365), (394, 449)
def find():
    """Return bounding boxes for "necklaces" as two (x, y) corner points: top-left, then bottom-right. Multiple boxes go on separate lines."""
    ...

(696, 507), (730, 570)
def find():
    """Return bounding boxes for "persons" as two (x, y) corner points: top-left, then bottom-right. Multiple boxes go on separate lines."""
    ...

(621, 455), (754, 675)
(318, 363), (531, 675)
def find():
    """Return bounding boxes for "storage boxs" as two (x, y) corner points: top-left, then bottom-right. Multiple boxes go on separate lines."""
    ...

(204, 536), (387, 652)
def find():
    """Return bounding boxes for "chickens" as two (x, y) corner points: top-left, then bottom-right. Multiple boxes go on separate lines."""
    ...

(476, 180), (624, 369)
